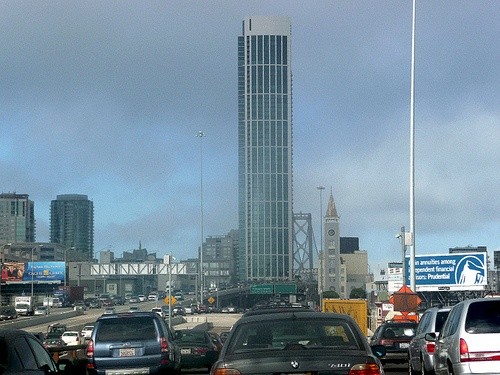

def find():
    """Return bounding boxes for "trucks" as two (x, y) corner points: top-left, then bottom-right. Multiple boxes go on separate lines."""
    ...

(165, 280), (177, 292)
(15, 296), (37, 317)
(54, 286), (84, 305)
(43, 297), (63, 307)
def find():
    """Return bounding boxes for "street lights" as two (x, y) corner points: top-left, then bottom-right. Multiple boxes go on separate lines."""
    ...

(31, 244), (44, 296)
(64, 247), (76, 290)
(395, 233), (406, 285)
(195, 131), (207, 305)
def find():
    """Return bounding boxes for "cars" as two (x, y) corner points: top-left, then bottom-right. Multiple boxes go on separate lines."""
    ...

(72, 280), (250, 316)
(176, 328), (224, 371)
(46, 324), (66, 336)
(34, 305), (50, 316)
(220, 331), (230, 343)
(0, 305), (18, 320)
(0, 326), (62, 375)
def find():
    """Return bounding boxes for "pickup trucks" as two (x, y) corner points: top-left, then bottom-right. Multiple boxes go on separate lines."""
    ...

(62, 330), (82, 345)
(43, 333), (64, 349)
(81, 326), (95, 339)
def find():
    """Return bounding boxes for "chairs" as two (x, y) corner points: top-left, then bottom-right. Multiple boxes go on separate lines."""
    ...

(319, 336), (344, 346)
(248, 335), (273, 347)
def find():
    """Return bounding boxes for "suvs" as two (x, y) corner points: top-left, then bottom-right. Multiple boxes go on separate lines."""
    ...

(371, 317), (417, 367)
(88, 311), (180, 375)
(408, 297), (500, 375)
(211, 302), (387, 375)
